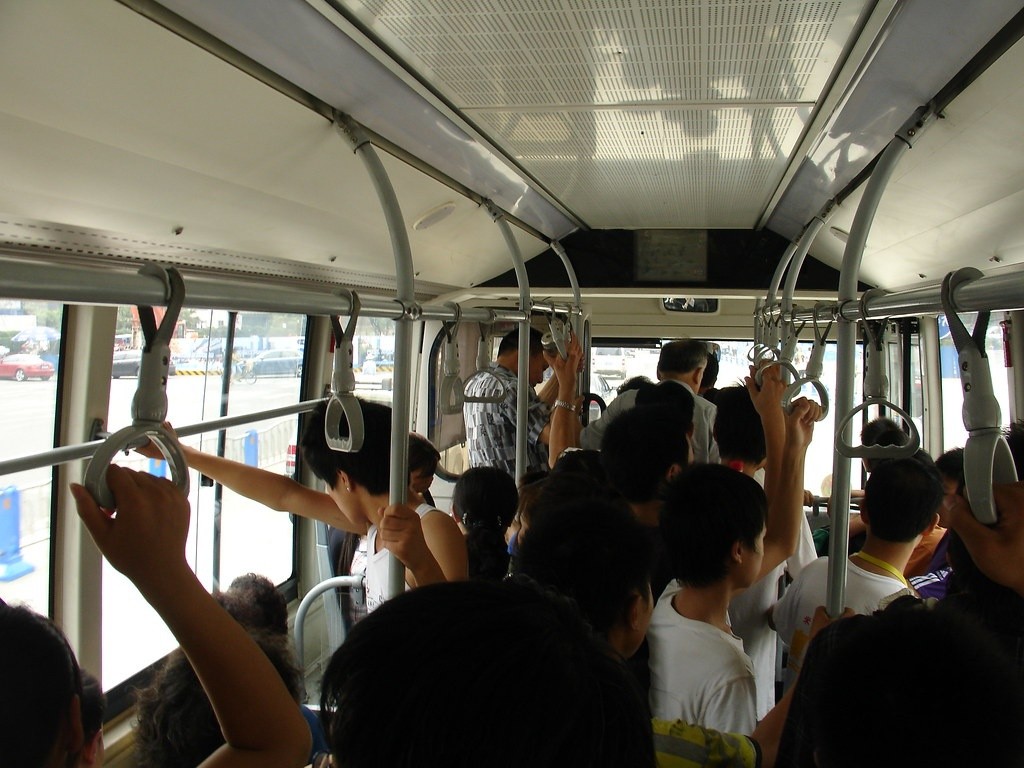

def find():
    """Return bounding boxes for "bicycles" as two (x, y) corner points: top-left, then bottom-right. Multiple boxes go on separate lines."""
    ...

(221, 363), (259, 386)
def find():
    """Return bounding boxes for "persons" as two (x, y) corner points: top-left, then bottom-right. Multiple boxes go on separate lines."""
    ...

(0, 326), (1024, 768)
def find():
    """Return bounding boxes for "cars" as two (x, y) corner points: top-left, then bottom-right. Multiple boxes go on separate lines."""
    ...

(624, 348), (663, 358)
(354, 352), (394, 370)
(112, 350), (177, 380)
(590, 373), (619, 420)
(0, 353), (55, 382)
(234, 349), (304, 377)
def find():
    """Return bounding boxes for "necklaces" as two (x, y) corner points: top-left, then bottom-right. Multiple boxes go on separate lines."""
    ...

(849, 551), (907, 587)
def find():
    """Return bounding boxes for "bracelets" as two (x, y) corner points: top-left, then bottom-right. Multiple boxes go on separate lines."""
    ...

(555, 399), (580, 413)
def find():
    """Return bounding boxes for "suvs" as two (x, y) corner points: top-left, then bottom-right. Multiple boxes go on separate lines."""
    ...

(592, 347), (630, 381)
